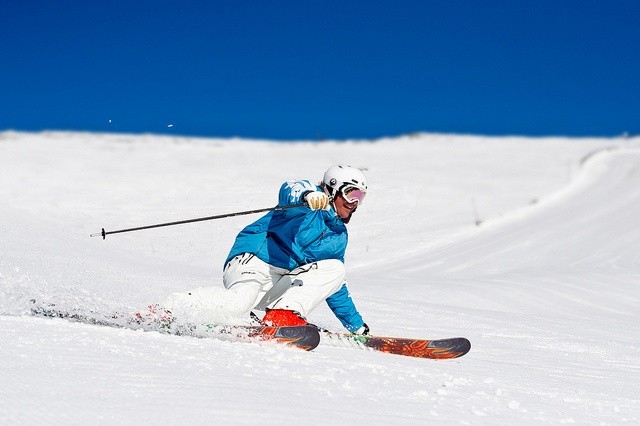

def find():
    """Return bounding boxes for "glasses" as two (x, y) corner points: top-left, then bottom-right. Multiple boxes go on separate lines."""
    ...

(341, 184), (366, 205)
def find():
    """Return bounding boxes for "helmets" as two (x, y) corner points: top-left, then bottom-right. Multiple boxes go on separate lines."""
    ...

(323, 165), (367, 213)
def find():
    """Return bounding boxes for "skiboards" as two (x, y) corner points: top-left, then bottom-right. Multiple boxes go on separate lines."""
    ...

(26, 296), (471, 358)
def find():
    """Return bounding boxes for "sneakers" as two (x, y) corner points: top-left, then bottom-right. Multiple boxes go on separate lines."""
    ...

(261, 310), (331, 332)
(133, 304), (175, 322)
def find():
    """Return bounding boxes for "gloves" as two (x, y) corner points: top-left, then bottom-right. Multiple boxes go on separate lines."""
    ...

(353, 324), (370, 336)
(304, 191), (334, 211)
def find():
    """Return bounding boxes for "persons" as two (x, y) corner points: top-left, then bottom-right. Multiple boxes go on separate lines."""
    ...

(129, 163), (369, 336)
